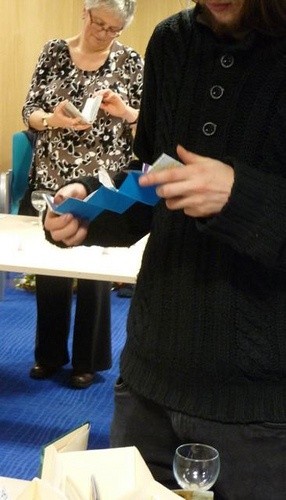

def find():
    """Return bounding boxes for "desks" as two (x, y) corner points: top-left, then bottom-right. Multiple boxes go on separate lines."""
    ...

(0, 214), (150, 283)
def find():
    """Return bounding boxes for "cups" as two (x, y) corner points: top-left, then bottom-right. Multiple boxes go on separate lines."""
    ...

(173, 443), (220, 491)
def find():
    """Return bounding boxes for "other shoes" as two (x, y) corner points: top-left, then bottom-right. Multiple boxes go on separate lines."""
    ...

(71, 371), (97, 389)
(30, 364), (63, 380)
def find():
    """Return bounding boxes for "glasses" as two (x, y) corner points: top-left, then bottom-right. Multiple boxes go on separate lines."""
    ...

(89, 9), (119, 39)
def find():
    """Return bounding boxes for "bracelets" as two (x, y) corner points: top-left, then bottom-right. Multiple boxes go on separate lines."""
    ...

(128, 117), (138, 125)
(41, 112), (58, 130)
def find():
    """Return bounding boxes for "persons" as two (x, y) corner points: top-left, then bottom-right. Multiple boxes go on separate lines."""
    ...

(21, 1), (144, 390)
(42, 0), (286, 500)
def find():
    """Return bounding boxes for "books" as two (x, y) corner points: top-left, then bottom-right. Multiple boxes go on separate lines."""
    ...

(54, 95), (103, 125)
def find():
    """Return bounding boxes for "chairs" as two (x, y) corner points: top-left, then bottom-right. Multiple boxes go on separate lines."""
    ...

(9, 131), (33, 215)
